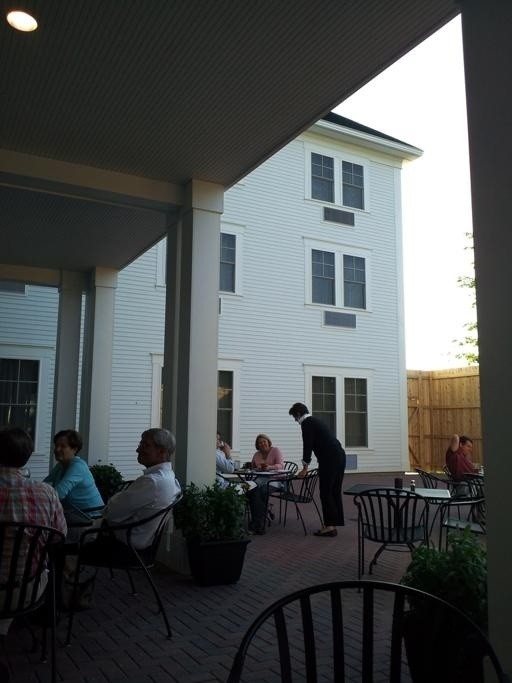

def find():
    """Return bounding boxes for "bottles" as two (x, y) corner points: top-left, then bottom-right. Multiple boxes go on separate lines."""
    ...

(410, 478), (416, 493)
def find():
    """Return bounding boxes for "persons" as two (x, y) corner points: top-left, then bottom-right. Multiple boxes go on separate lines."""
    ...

(248, 432), (288, 535)
(288, 401), (347, 538)
(1, 425), (69, 657)
(40, 428), (108, 545)
(55, 426), (182, 612)
(214, 431), (246, 501)
(445, 432), (486, 524)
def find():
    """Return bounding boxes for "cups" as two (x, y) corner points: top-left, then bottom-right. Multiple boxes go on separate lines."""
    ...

(395, 477), (404, 493)
(234, 459), (241, 473)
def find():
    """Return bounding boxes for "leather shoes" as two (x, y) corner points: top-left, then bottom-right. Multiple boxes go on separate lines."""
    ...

(313, 528), (338, 538)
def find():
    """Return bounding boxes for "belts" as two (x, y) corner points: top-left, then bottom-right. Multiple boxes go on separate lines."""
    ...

(0, 576), (34, 591)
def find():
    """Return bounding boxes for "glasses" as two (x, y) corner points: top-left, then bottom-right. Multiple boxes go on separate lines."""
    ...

(138, 441), (156, 449)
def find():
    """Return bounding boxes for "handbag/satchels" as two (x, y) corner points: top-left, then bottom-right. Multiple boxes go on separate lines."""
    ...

(61, 576), (96, 612)
